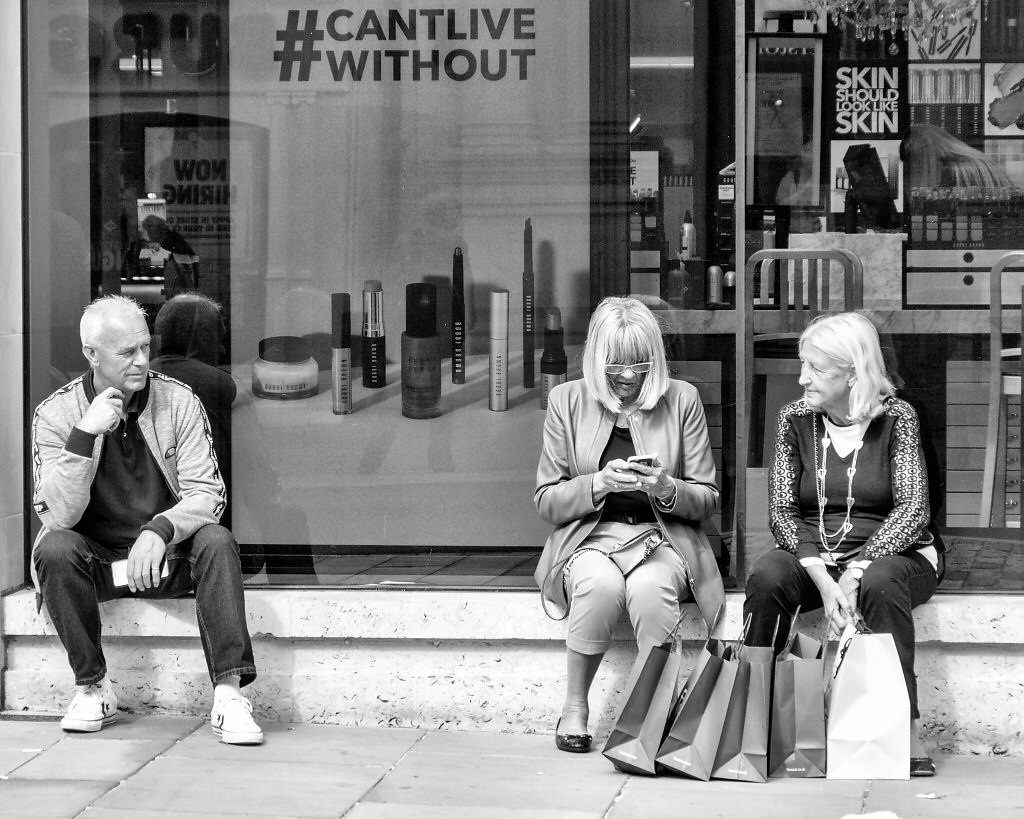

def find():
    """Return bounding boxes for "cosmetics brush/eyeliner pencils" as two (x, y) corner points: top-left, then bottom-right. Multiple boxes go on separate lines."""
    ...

(522, 218), (534, 388)
(451, 247), (466, 384)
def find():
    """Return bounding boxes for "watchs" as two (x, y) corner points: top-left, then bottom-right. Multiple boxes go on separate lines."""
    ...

(850, 567), (864, 580)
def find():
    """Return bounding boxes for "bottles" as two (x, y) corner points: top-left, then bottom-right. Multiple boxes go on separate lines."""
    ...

(706, 250), (723, 303)
(679, 210), (696, 260)
(631, 188), (658, 250)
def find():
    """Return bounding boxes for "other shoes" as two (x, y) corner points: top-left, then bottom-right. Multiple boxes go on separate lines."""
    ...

(556, 716), (592, 752)
(910, 757), (936, 776)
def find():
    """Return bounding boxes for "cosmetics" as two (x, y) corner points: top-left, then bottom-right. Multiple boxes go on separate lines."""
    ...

(906, 0), (1024, 135)
(629, 188), (1024, 305)
(251, 282), (508, 419)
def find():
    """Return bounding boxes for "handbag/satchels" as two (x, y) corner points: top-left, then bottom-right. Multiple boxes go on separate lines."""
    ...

(607, 527), (664, 576)
(601, 607), (688, 777)
(654, 603), (752, 782)
(711, 613), (781, 783)
(825, 619), (911, 781)
(768, 605), (835, 779)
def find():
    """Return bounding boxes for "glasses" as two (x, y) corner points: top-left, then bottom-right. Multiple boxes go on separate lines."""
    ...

(605, 361), (654, 375)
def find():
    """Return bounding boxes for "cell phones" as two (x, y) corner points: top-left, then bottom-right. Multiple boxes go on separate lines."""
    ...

(110, 552), (170, 587)
(628, 455), (653, 476)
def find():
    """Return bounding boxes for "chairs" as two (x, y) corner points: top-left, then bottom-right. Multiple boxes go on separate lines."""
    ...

(744, 248), (864, 490)
(980, 250), (1024, 528)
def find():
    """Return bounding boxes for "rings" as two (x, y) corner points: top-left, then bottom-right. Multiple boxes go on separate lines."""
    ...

(614, 481), (618, 488)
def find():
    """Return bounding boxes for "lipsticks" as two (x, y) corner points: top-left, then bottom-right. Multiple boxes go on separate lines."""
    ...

(360, 280), (386, 387)
(540, 305), (567, 410)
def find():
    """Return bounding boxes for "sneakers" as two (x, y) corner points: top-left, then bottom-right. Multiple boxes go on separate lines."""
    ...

(61, 680), (119, 732)
(211, 696), (263, 744)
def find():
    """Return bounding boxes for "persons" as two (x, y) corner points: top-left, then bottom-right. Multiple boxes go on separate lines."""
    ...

(741, 314), (945, 773)
(775, 136), (814, 204)
(899, 123), (1010, 186)
(149, 292), (320, 585)
(533, 296), (725, 748)
(30, 294), (263, 745)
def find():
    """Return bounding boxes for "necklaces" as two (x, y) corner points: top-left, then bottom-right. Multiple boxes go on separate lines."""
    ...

(621, 423), (624, 426)
(812, 409), (863, 551)
